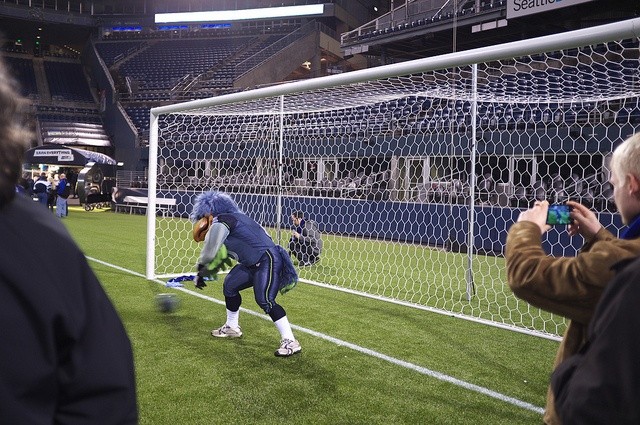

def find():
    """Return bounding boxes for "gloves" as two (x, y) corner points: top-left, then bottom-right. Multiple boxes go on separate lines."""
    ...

(193, 274), (207, 291)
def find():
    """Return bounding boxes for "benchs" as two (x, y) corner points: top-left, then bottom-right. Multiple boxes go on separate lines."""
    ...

(116, 196), (177, 217)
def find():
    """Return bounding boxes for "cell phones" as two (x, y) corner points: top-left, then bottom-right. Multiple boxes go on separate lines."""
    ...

(545, 201), (578, 226)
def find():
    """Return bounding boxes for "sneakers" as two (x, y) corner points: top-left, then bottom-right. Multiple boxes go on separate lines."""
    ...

(210, 323), (242, 338)
(274, 338), (302, 357)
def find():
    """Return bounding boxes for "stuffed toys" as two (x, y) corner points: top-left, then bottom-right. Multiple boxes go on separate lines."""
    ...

(188, 190), (302, 357)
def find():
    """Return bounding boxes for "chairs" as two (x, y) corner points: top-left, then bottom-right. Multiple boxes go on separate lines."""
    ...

(45, 41), (83, 58)
(347, 0), (506, 42)
(177, 34), (303, 70)
(109, 37), (178, 70)
(43, 60), (95, 102)
(0, 55), (38, 96)
(103, 25), (300, 40)
(37, 106), (115, 124)
(95, 41), (150, 67)
(0, 47), (31, 54)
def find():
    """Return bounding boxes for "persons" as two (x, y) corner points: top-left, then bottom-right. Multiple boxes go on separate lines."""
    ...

(0, 59), (139, 425)
(553, 206), (563, 224)
(503, 131), (639, 425)
(15, 172), (70, 217)
(550, 251), (639, 425)
(287, 209), (322, 269)
